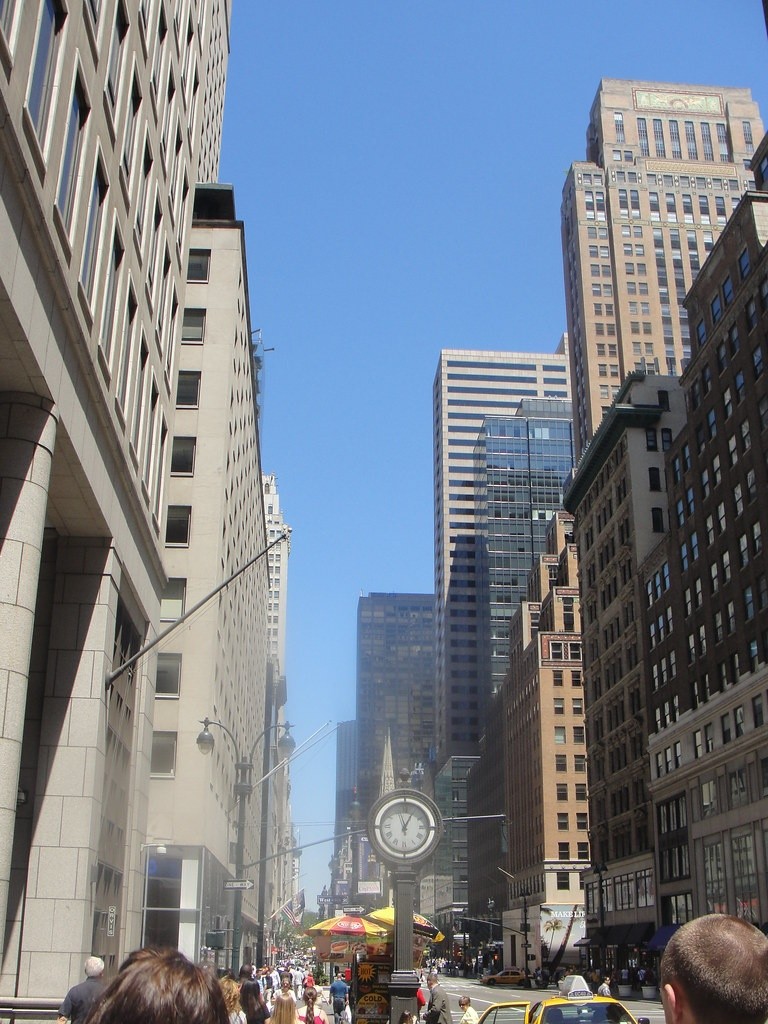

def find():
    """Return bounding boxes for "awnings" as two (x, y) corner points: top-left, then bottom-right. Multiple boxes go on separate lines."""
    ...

(648, 924), (685, 950)
(590, 922), (656, 945)
(574, 938), (591, 947)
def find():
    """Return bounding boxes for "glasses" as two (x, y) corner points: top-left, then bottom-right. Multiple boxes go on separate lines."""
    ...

(459, 1004), (466, 1006)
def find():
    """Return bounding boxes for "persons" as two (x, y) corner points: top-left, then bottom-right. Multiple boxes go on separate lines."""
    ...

(620, 966), (630, 985)
(398, 1009), (413, 1024)
(423, 974), (453, 1024)
(223, 951), (325, 1009)
(239, 978), (271, 1024)
(57, 956), (106, 1024)
(636, 965), (649, 989)
(218, 977), (247, 1024)
(425, 957), (602, 994)
(596, 976), (615, 997)
(663, 913), (768, 1024)
(270, 995), (296, 1024)
(417, 987), (426, 1022)
(328, 972), (349, 1024)
(82, 944), (231, 1024)
(458, 996), (480, 1024)
(294, 986), (329, 1024)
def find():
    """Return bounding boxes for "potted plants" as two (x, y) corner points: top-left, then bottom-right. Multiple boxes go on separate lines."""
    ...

(556, 967), (657, 999)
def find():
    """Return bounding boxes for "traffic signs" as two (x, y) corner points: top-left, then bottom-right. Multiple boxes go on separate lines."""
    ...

(342, 906), (366, 915)
(223, 880), (255, 890)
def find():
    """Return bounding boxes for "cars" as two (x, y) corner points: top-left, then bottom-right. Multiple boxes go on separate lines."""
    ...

(478, 975), (650, 1024)
(481, 971), (526, 986)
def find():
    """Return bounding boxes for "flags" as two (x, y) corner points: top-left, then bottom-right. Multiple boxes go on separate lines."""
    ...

(282, 888), (306, 927)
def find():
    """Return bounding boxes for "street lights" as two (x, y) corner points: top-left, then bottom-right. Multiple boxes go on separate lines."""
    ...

(506, 876), (531, 989)
(196, 717), (296, 979)
(462, 906), (468, 978)
(329, 855), (340, 985)
(485, 897), (495, 967)
(348, 800), (360, 906)
(593, 862), (609, 983)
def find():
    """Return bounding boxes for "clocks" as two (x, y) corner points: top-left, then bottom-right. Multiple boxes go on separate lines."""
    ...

(364, 789), (445, 867)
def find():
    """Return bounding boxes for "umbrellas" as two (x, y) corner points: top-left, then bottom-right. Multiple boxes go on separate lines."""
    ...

(365, 905), (446, 943)
(307, 913), (388, 937)
